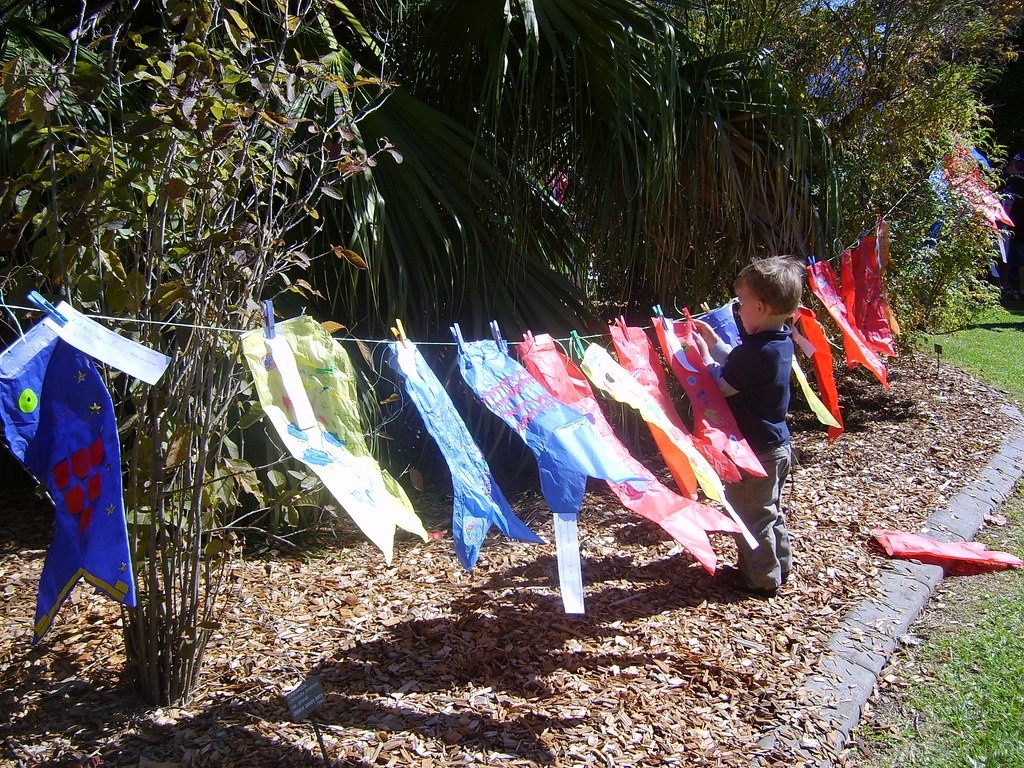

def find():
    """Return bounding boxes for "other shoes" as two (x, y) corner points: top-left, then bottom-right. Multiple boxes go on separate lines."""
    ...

(781, 571), (789, 584)
(727, 568), (776, 598)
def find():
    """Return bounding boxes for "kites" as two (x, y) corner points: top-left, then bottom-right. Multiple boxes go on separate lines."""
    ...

(0, 127), (1024, 644)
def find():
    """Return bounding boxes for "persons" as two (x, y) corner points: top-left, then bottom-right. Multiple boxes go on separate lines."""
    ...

(1000, 153), (1024, 302)
(690, 256), (802, 597)
